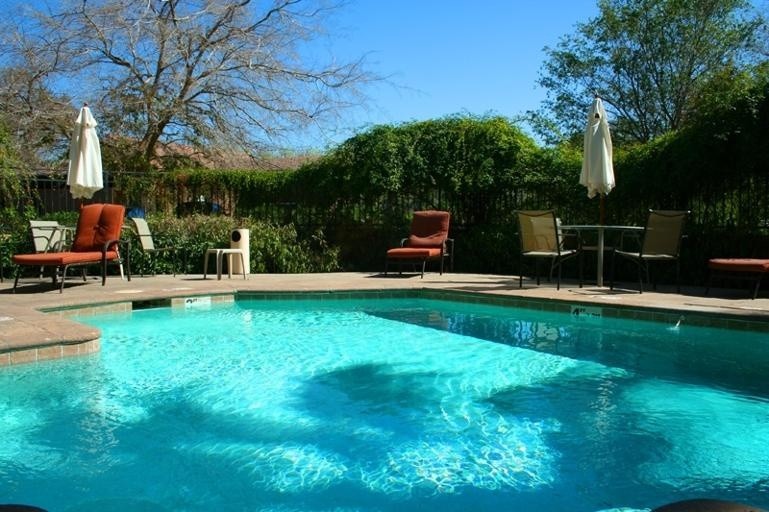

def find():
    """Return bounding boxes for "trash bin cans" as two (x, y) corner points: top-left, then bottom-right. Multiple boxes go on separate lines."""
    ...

(231, 229), (250, 275)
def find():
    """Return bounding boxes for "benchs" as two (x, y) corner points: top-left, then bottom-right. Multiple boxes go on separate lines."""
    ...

(703, 258), (769, 300)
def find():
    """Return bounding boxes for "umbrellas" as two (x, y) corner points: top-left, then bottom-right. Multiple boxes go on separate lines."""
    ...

(578, 93), (617, 225)
(66, 101), (104, 209)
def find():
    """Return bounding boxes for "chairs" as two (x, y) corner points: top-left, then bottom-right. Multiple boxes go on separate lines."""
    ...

(12, 203), (130, 294)
(131, 218), (187, 277)
(384, 210), (454, 279)
(514, 210), (579, 291)
(610, 209), (692, 294)
(28, 220), (87, 282)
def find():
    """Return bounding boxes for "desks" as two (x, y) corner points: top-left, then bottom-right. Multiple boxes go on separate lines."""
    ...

(557, 225), (645, 295)
(55, 226), (131, 280)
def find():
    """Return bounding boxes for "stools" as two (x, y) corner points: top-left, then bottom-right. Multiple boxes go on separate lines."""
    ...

(203, 249), (247, 281)
